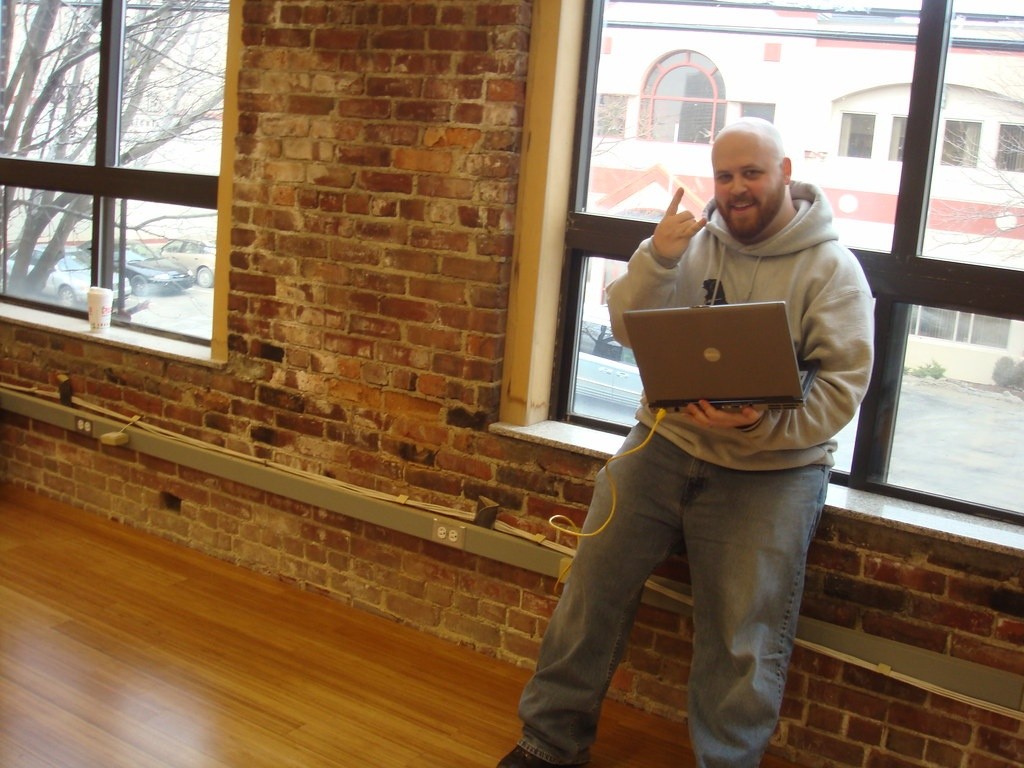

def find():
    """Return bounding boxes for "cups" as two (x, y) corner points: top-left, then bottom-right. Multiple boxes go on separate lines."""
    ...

(88, 286), (113, 333)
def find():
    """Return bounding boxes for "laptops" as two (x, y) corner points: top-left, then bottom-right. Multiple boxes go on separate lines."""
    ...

(624, 301), (821, 409)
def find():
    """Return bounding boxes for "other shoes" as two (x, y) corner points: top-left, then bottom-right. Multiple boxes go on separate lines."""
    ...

(497, 745), (558, 768)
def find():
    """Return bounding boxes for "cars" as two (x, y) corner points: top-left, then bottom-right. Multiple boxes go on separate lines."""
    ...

(156, 238), (216, 288)
(74, 239), (196, 298)
(571, 309), (653, 421)
(0, 241), (132, 303)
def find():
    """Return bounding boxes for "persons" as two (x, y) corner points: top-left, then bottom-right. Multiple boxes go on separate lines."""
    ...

(495, 116), (876, 768)
